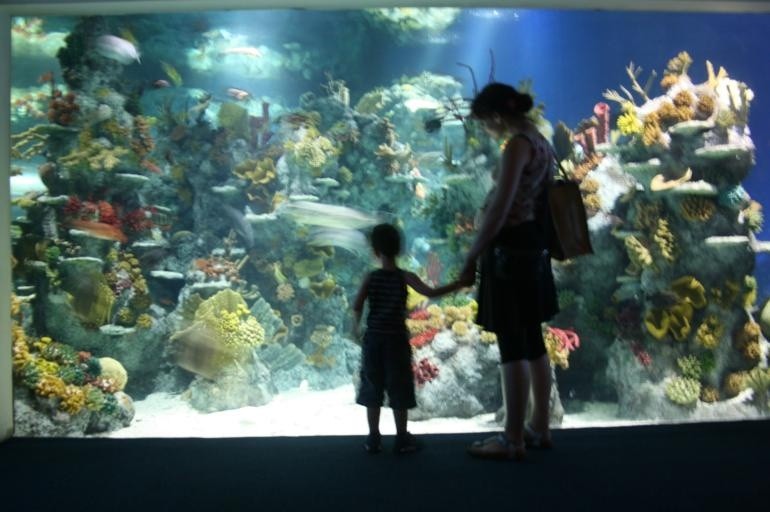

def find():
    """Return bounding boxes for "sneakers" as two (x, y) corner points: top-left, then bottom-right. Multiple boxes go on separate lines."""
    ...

(364, 435), (384, 454)
(393, 436), (424, 455)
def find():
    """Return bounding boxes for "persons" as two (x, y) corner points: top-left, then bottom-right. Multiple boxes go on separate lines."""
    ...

(452, 79), (562, 464)
(350, 220), (477, 454)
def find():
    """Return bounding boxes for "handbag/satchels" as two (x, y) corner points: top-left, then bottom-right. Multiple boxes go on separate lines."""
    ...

(540, 137), (596, 262)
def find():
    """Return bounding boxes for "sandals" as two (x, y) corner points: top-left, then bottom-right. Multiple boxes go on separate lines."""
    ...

(523, 424), (552, 449)
(466, 433), (527, 463)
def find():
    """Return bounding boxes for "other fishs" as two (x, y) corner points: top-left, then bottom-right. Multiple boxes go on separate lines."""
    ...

(304, 352), (336, 369)
(18, 34), (428, 351)
